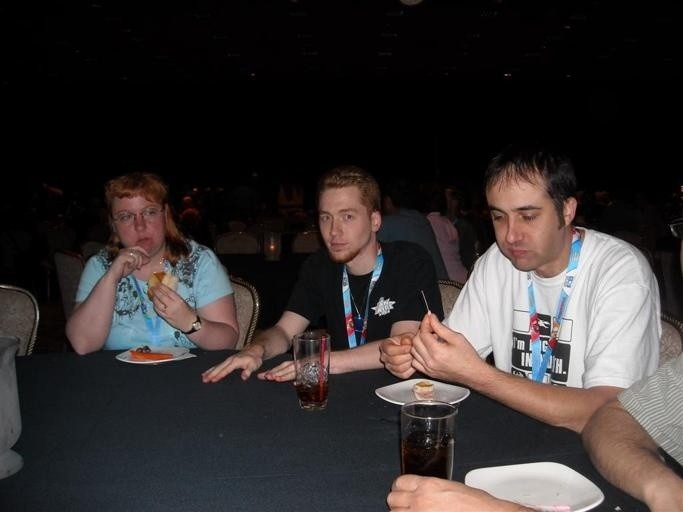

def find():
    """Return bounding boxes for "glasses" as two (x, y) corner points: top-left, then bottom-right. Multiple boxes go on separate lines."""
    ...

(112, 208), (163, 222)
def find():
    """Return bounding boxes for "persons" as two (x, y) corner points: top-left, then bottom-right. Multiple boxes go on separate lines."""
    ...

(377, 142), (661, 434)
(580, 353), (682, 511)
(1, 151), (682, 318)
(199, 170), (444, 385)
(65, 171), (242, 357)
(387, 474), (540, 512)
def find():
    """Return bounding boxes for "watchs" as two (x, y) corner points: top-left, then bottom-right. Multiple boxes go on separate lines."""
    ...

(181, 314), (202, 335)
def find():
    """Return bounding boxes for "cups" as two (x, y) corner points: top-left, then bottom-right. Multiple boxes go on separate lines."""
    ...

(263, 232), (282, 262)
(399, 400), (458, 480)
(292, 330), (331, 412)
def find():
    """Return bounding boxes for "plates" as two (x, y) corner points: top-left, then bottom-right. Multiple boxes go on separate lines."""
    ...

(374, 378), (470, 406)
(114, 346), (189, 365)
(463, 462), (604, 512)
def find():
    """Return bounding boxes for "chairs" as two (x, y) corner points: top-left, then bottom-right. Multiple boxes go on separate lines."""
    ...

(1, 283), (41, 364)
(226, 277), (261, 352)
